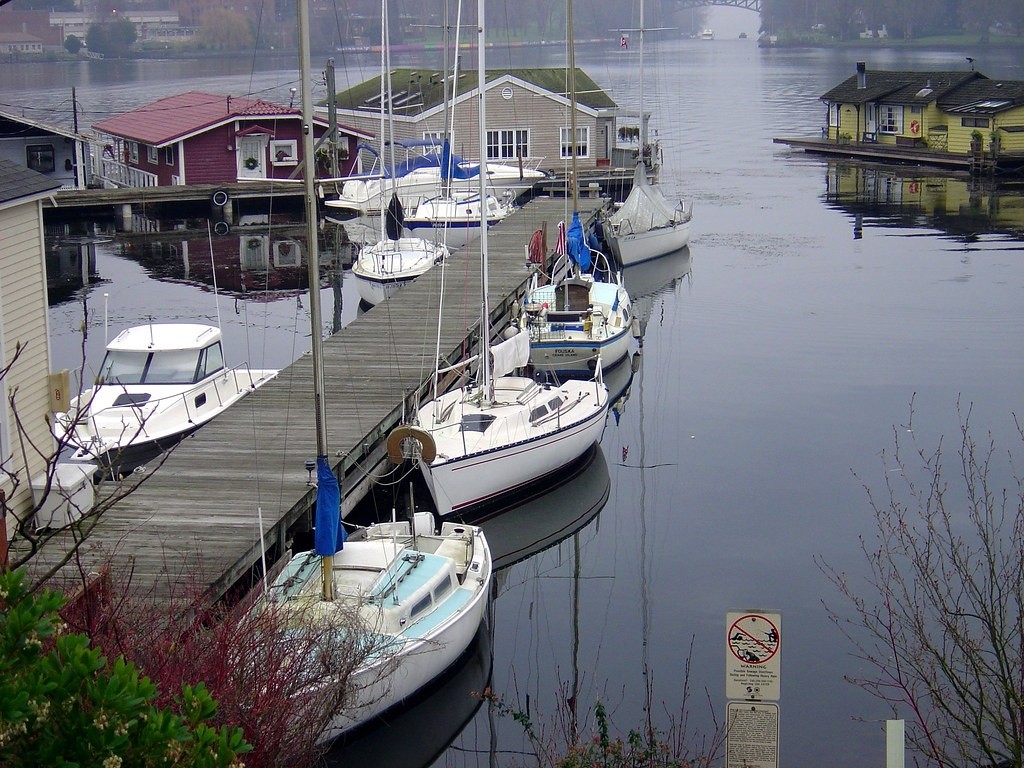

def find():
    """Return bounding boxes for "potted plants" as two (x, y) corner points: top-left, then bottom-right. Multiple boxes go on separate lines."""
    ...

(839, 133), (852, 144)
(989, 130), (1002, 143)
(970, 129), (983, 141)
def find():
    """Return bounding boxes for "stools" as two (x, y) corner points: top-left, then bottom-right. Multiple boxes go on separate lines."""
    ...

(26, 462), (98, 529)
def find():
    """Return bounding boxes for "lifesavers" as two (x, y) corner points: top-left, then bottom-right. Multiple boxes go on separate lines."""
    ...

(214, 221), (230, 236)
(210, 189), (229, 208)
(385, 424), (437, 466)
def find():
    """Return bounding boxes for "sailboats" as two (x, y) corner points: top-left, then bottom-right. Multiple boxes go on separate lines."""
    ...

(399, 0), (611, 523)
(501, 0), (637, 382)
(318, 0), (544, 312)
(426, 441), (610, 767)
(594, 0), (693, 269)
(219, 0), (493, 760)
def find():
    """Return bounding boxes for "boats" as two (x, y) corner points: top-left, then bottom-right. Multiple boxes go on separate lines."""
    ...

(701, 30), (714, 40)
(54, 290), (284, 468)
